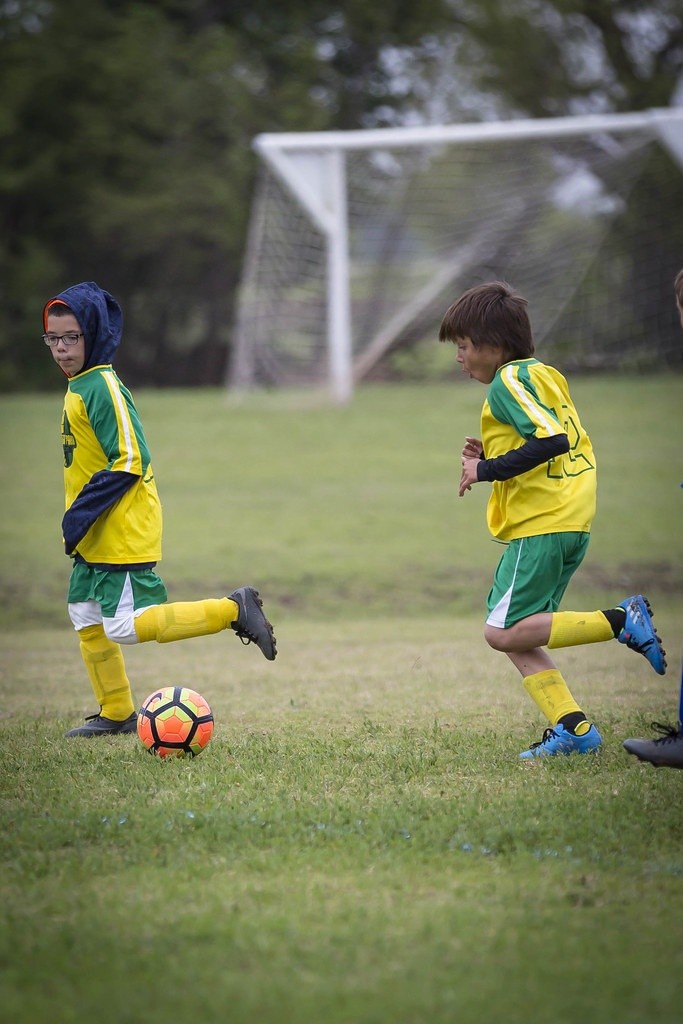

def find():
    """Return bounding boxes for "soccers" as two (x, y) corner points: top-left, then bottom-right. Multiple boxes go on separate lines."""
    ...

(137, 686), (215, 758)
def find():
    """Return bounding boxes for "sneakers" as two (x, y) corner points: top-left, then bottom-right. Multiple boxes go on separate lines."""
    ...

(615, 593), (667, 675)
(623, 722), (683, 770)
(226, 586), (278, 661)
(515, 723), (603, 761)
(63, 711), (138, 739)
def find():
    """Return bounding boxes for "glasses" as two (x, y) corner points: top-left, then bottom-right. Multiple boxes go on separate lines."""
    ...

(41, 333), (84, 347)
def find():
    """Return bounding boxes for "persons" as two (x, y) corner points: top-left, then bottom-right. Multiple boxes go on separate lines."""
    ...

(438, 281), (668, 762)
(42, 283), (278, 738)
